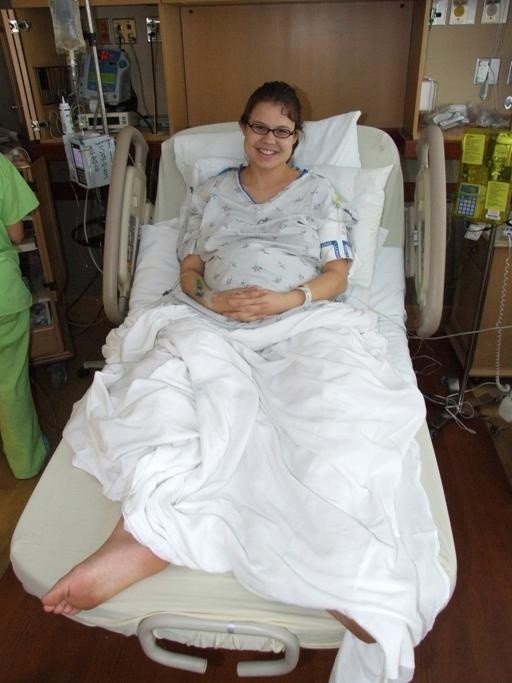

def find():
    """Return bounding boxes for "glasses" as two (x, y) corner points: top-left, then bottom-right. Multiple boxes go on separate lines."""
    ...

(247, 121), (296, 139)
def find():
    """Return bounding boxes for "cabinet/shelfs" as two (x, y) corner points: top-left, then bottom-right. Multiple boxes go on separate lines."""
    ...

(445, 214), (512, 378)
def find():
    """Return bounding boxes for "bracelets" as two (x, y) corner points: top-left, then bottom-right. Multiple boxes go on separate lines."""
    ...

(292, 288), (312, 310)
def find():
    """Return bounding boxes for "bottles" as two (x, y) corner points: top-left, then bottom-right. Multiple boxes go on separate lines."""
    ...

(60, 94), (76, 135)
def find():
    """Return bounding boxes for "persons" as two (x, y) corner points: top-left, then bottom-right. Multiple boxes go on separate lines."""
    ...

(1, 154), (51, 479)
(40, 80), (378, 644)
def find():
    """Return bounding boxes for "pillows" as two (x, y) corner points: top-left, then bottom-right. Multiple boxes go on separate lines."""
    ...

(192, 159), (395, 287)
(173, 111), (368, 186)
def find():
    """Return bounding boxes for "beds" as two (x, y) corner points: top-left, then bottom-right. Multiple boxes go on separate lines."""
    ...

(8, 121), (459, 679)
